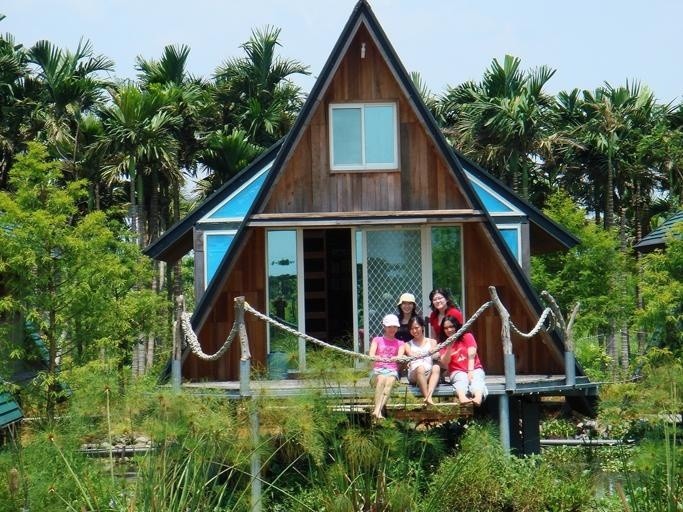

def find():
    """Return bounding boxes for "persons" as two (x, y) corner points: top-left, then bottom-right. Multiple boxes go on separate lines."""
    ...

(426, 287), (465, 341)
(367, 312), (406, 420)
(433, 313), (489, 407)
(403, 313), (441, 409)
(394, 292), (425, 340)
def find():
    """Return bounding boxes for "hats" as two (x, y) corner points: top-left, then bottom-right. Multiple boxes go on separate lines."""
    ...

(383, 314), (400, 328)
(396, 293), (417, 306)
